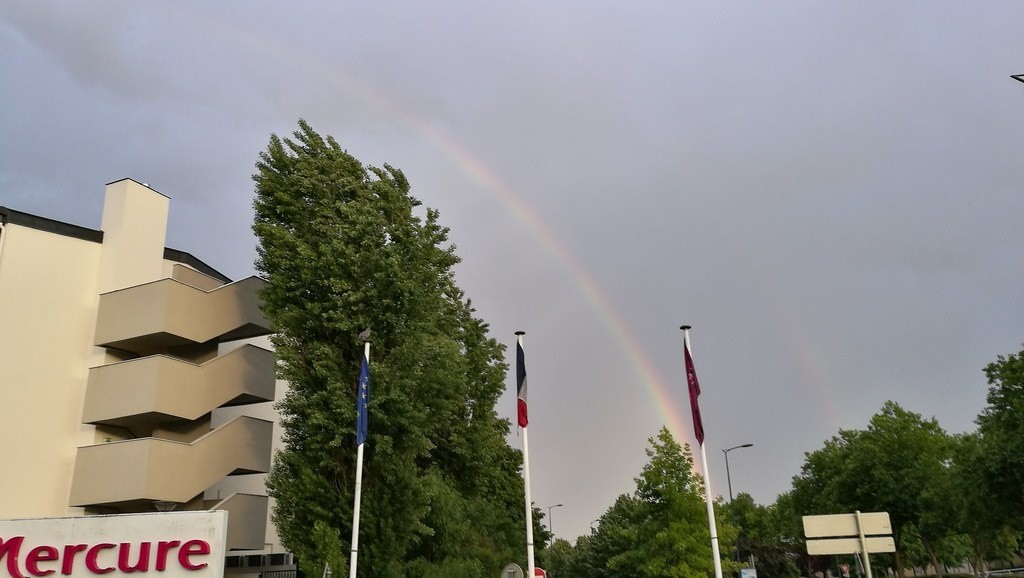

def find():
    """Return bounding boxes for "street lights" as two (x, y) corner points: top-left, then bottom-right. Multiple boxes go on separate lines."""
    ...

(548, 504), (563, 547)
(591, 519), (598, 536)
(721, 444), (753, 563)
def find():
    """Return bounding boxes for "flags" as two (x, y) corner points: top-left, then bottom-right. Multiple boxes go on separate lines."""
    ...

(516, 340), (528, 435)
(684, 339), (705, 445)
(357, 356), (370, 444)
